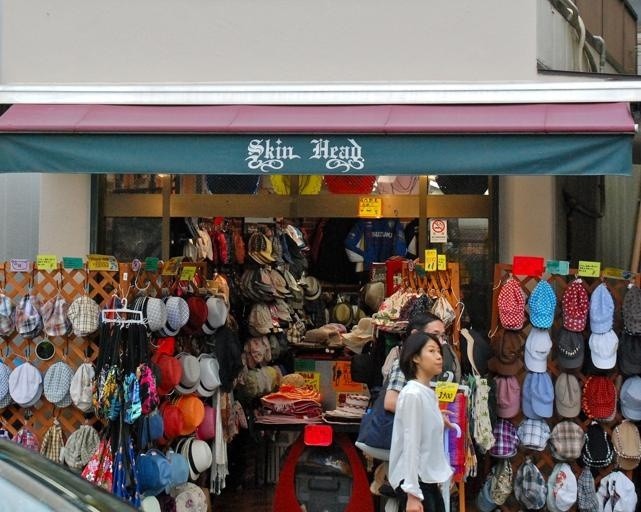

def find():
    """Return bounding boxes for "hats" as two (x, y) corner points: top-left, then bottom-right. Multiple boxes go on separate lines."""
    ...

(8, 362), (43, 408)
(0, 294), (16, 335)
(44, 362), (75, 408)
(0, 425), (101, 468)
(15, 294), (44, 339)
(476, 277), (640, 511)
(67, 296), (101, 337)
(40, 294), (71, 337)
(0, 362), (12, 408)
(70, 363), (94, 412)
(80, 291), (227, 512)
(239, 223), (385, 424)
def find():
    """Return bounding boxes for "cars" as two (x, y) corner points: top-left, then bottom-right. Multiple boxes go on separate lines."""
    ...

(0, 433), (149, 512)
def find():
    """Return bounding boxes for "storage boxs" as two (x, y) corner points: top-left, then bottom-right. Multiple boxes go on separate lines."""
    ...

(294, 464), (351, 512)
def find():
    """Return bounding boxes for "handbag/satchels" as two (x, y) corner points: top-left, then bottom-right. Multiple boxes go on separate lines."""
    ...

(354, 413), (395, 462)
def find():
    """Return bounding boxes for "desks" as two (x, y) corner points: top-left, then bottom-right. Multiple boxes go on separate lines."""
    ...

(253, 420), (360, 512)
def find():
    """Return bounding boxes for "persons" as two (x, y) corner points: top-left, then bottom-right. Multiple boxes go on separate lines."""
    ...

(387, 328), (452, 511)
(372, 311), (461, 429)
